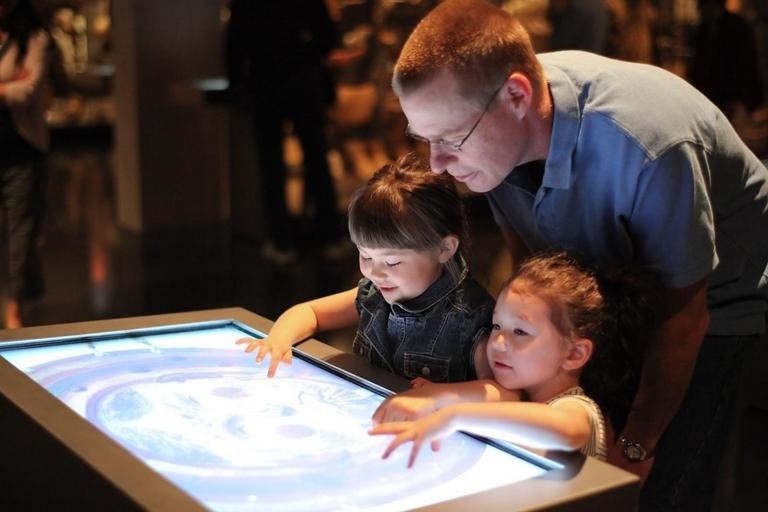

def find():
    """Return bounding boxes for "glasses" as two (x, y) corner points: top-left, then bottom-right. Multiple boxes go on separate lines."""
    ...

(404, 91), (503, 155)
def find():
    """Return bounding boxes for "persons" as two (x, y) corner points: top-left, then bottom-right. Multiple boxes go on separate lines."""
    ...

(1, 1), (52, 330)
(221, 0), (341, 251)
(544, 0), (768, 129)
(367, 249), (605, 467)
(394, 1), (768, 510)
(235, 152), (497, 390)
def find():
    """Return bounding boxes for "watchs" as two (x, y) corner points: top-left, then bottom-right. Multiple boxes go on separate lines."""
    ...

(613, 435), (660, 462)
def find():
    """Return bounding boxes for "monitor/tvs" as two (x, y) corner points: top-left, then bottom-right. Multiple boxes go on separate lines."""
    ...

(0, 322), (565, 512)
